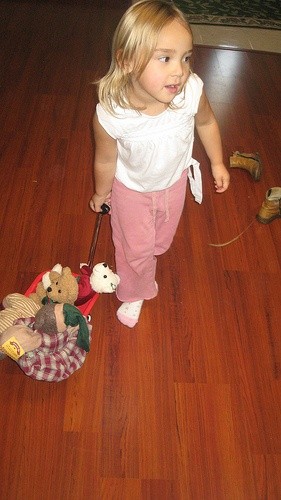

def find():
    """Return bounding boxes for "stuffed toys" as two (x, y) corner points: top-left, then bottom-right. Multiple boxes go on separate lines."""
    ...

(29, 263), (79, 305)
(12, 303), (93, 382)
(74, 262), (120, 306)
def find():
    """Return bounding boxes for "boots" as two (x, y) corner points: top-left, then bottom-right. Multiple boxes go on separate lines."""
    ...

(257, 187), (281, 224)
(229, 151), (260, 180)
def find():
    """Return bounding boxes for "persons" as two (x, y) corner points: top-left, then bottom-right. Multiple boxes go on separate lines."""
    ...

(89, 0), (230, 329)
